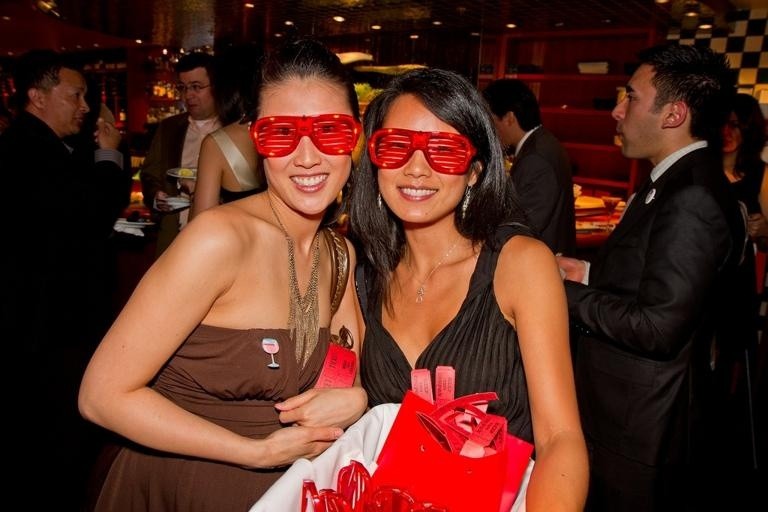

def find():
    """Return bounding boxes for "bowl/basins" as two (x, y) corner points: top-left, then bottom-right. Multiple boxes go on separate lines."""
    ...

(577, 62), (609, 74)
(505, 64), (545, 75)
(624, 62), (641, 75)
(592, 98), (616, 111)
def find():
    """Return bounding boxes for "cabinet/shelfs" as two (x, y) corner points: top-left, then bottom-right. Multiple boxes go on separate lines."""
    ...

(476, 27), (667, 201)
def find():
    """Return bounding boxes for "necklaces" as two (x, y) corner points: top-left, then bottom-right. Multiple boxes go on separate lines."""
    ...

(269, 202), (321, 367)
(405, 234), (466, 304)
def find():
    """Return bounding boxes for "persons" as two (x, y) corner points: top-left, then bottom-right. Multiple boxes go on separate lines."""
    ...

(353, 68), (590, 511)
(76, 40), (369, 512)
(1, 53), (129, 512)
(479, 78), (577, 261)
(553, 40), (737, 511)
(725, 92), (768, 309)
(176, 45), (268, 225)
(141, 51), (219, 264)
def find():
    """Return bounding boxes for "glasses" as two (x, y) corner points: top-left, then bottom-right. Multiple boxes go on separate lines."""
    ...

(365, 127), (479, 175)
(248, 113), (362, 160)
(175, 83), (211, 92)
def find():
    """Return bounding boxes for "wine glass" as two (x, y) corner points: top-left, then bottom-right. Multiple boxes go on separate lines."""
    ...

(600, 196), (624, 235)
(262, 337), (280, 369)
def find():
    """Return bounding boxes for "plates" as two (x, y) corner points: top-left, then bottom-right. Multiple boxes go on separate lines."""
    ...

(116, 218), (156, 229)
(165, 167), (197, 180)
(575, 226), (605, 235)
